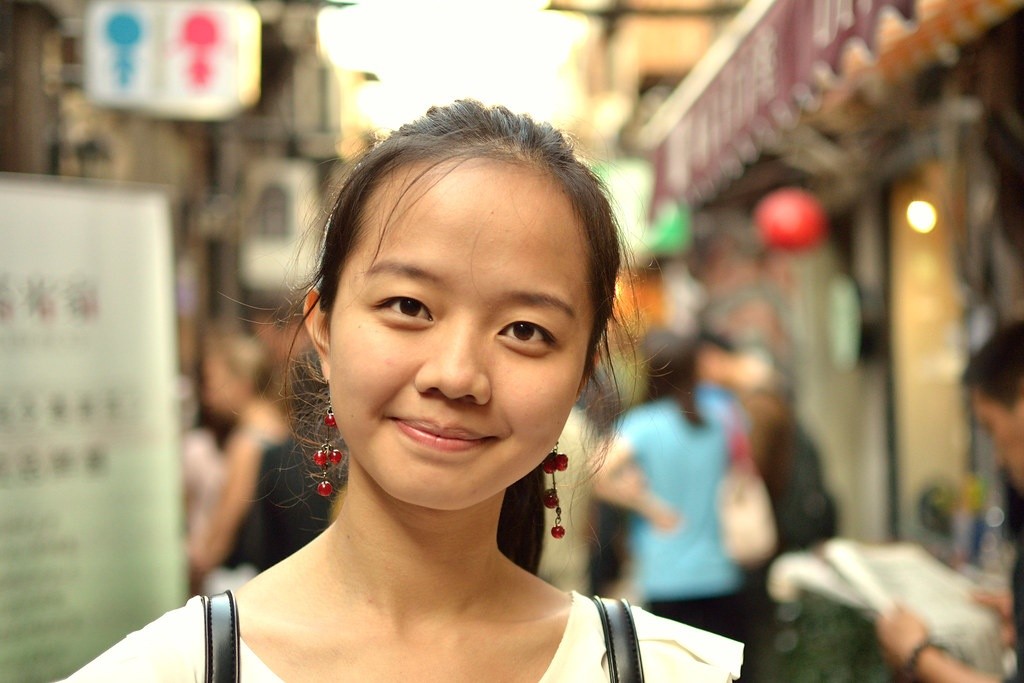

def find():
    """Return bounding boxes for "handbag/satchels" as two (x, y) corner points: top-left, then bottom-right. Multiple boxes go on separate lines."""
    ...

(717, 404), (776, 566)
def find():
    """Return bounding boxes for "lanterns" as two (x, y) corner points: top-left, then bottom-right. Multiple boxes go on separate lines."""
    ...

(757, 184), (830, 255)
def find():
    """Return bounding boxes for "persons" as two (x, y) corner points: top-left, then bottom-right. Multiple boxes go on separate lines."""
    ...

(43, 98), (742, 680)
(180, 292), (1024, 681)
